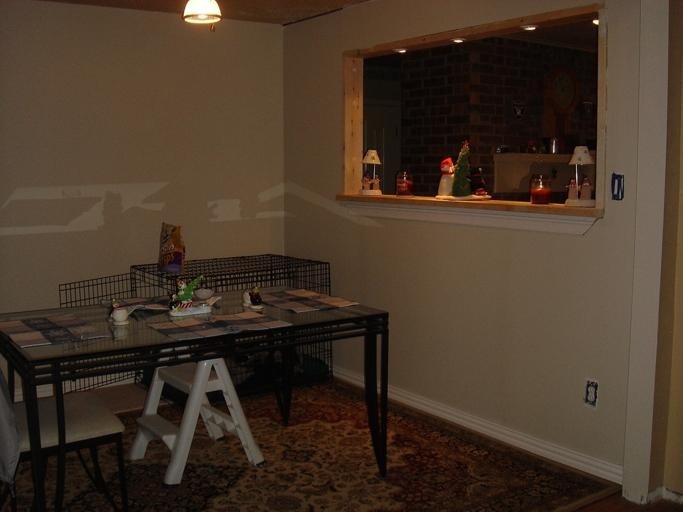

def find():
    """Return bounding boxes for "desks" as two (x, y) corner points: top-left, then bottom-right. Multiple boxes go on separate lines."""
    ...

(494, 153), (594, 195)
(0, 288), (390, 512)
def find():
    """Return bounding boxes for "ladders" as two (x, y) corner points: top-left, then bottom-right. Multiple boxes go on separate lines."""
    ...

(129, 343), (265, 485)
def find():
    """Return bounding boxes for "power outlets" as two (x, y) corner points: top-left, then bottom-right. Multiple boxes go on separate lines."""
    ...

(585, 380), (598, 407)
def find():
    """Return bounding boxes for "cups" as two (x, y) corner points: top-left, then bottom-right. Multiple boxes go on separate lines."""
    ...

(110, 308), (129, 321)
(396, 171), (413, 195)
(530, 175), (552, 205)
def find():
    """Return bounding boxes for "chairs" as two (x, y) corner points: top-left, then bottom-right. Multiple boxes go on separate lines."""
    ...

(0, 367), (127, 512)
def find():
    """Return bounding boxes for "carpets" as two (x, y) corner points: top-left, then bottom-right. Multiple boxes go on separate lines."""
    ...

(0, 377), (622, 512)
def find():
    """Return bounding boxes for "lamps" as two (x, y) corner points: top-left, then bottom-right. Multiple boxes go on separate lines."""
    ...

(182, 0), (223, 33)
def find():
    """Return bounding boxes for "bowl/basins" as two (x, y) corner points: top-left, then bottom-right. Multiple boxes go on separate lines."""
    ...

(194, 288), (214, 299)
